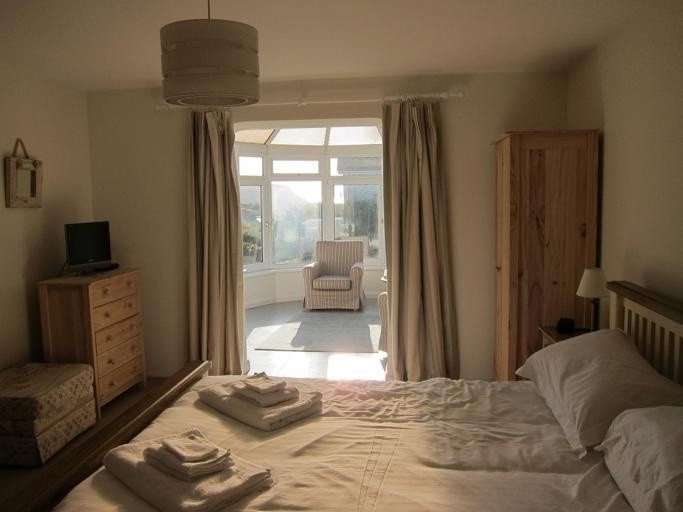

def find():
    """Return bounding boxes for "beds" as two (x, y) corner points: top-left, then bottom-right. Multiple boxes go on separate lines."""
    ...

(31, 281), (683, 512)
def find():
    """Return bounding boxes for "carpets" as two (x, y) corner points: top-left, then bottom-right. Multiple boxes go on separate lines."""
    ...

(254, 309), (383, 353)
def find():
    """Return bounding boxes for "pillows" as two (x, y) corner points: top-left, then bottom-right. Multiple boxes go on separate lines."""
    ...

(513, 326), (683, 511)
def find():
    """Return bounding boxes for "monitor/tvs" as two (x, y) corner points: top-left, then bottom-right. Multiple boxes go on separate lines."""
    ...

(62, 220), (114, 265)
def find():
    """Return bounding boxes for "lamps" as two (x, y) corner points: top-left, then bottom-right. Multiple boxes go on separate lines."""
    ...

(160, 0), (260, 109)
(576, 267), (607, 332)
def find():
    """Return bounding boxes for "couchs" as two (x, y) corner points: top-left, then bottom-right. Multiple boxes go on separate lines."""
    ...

(303, 240), (364, 312)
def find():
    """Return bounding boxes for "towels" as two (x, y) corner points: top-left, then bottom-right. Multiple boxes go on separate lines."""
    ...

(197, 368), (323, 429)
(102, 429), (274, 512)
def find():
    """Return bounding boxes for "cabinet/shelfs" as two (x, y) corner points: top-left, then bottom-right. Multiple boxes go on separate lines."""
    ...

(491, 128), (601, 381)
(35, 267), (148, 419)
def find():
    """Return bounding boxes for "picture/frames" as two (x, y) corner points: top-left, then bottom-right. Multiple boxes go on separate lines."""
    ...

(5, 156), (42, 208)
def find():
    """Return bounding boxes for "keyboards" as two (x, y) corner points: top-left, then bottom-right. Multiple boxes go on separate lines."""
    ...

(70, 262), (119, 276)
